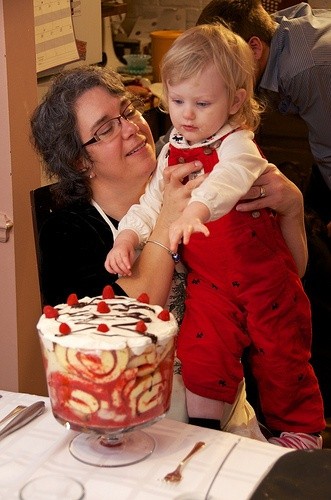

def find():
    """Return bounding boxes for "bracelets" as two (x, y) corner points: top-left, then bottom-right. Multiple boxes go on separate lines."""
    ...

(146, 239), (180, 263)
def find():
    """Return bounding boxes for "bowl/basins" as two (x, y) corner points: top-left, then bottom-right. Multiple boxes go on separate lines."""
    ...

(123, 54), (151, 69)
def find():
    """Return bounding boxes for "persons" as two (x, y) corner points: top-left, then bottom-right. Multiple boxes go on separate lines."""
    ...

(105, 23), (327, 451)
(32, 66), (266, 446)
(195, 0), (331, 244)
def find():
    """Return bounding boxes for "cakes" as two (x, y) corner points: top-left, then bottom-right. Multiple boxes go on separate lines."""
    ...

(35, 285), (178, 430)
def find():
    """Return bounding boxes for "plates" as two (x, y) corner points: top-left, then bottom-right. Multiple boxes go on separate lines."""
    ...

(118, 65), (152, 74)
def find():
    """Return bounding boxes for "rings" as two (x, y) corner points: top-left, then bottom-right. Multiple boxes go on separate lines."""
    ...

(260, 186), (266, 197)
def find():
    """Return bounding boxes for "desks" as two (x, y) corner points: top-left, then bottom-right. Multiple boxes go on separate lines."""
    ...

(0, 389), (298, 500)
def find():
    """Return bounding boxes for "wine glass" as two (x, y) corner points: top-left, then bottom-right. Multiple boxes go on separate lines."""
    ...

(36, 328), (175, 467)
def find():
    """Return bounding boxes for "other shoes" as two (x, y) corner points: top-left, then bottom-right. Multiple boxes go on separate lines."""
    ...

(269, 432), (323, 451)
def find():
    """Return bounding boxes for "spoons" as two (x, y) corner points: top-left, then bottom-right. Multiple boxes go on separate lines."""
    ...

(162, 442), (204, 482)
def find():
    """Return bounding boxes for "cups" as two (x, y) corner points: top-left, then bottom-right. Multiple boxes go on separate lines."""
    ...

(19, 474), (85, 500)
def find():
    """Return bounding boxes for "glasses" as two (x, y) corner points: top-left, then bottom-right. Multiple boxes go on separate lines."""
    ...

(78, 98), (146, 150)
